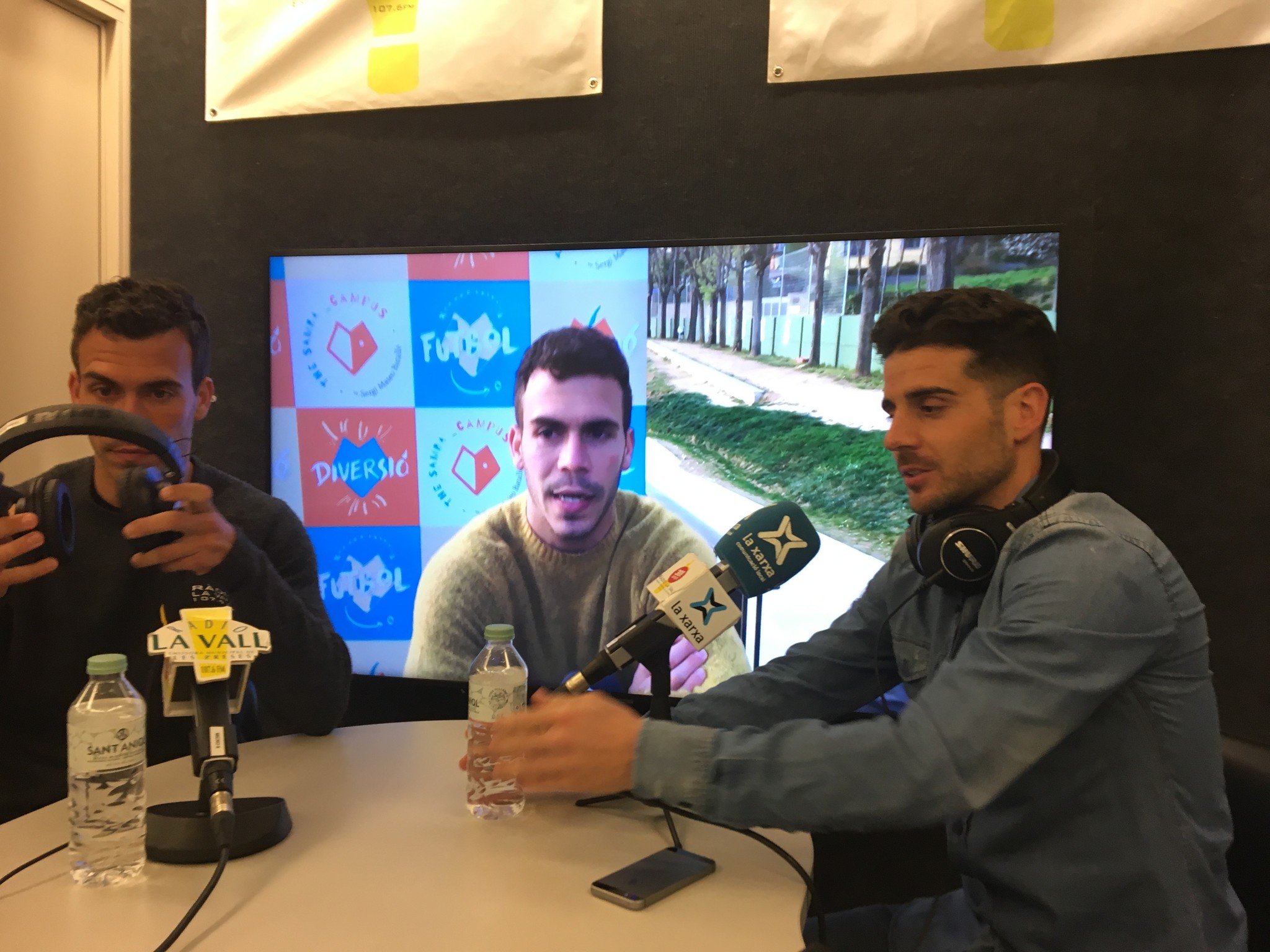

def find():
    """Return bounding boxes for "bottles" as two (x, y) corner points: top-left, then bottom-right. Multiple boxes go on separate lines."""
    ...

(67, 653), (147, 888)
(465, 624), (528, 820)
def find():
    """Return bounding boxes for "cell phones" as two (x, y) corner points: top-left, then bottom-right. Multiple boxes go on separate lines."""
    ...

(589, 846), (716, 911)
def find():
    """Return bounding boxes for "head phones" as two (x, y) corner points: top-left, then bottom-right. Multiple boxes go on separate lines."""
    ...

(1, 402), (190, 566)
(905, 447), (1069, 592)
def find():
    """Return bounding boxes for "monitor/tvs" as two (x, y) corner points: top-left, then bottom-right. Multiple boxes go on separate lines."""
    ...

(259, 220), (1070, 702)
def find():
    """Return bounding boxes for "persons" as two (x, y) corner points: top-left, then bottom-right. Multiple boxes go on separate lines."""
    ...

(459, 284), (1252, 952)
(401, 322), (752, 698)
(0, 275), (354, 827)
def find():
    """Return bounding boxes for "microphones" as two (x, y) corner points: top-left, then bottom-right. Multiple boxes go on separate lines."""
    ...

(560, 500), (822, 694)
(170, 574), (242, 851)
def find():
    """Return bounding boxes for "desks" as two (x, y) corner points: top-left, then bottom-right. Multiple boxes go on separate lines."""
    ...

(0, 715), (811, 952)
